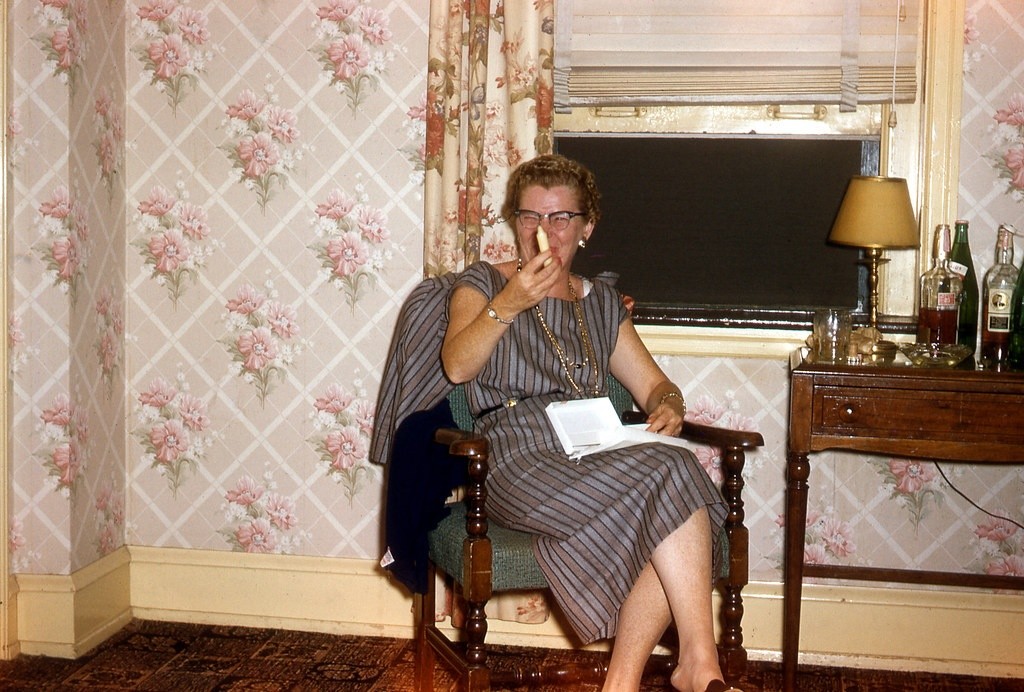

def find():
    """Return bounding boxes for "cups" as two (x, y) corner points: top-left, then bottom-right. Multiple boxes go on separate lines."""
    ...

(812, 308), (852, 364)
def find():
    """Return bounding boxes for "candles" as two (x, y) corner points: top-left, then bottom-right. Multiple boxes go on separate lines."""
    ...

(537, 225), (552, 268)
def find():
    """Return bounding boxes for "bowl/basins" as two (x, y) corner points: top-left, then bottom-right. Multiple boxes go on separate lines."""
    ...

(901, 344), (973, 368)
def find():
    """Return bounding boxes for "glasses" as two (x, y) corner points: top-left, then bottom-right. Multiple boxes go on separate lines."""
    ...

(514, 210), (586, 230)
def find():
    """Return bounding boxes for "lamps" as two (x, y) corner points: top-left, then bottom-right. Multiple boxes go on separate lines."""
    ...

(829, 175), (922, 355)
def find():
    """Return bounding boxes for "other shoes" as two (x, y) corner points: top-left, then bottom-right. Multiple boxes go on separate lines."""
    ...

(705, 679), (736, 692)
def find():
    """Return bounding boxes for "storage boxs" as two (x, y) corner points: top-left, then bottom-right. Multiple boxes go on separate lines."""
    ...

(544, 397), (623, 453)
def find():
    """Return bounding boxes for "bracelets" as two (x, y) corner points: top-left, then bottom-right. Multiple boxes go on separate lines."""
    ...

(660, 391), (687, 420)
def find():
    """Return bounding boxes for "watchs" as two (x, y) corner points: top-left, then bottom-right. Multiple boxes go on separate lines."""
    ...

(486, 300), (515, 325)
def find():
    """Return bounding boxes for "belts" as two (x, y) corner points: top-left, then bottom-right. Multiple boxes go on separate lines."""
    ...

(476, 391), (564, 419)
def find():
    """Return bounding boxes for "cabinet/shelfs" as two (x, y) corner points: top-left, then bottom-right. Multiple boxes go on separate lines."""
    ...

(782, 347), (1024, 692)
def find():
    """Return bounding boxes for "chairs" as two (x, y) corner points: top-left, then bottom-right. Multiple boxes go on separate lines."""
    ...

(394, 277), (765, 692)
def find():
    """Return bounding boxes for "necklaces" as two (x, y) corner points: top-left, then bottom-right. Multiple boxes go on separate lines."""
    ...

(516, 254), (602, 400)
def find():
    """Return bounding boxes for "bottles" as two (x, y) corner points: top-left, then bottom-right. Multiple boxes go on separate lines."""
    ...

(952, 220), (979, 369)
(1010, 260), (1024, 372)
(979, 225), (1021, 368)
(916, 225), (962, 343)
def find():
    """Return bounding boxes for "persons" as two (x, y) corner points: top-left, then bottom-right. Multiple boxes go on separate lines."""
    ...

(442, 154), (744, 692)
